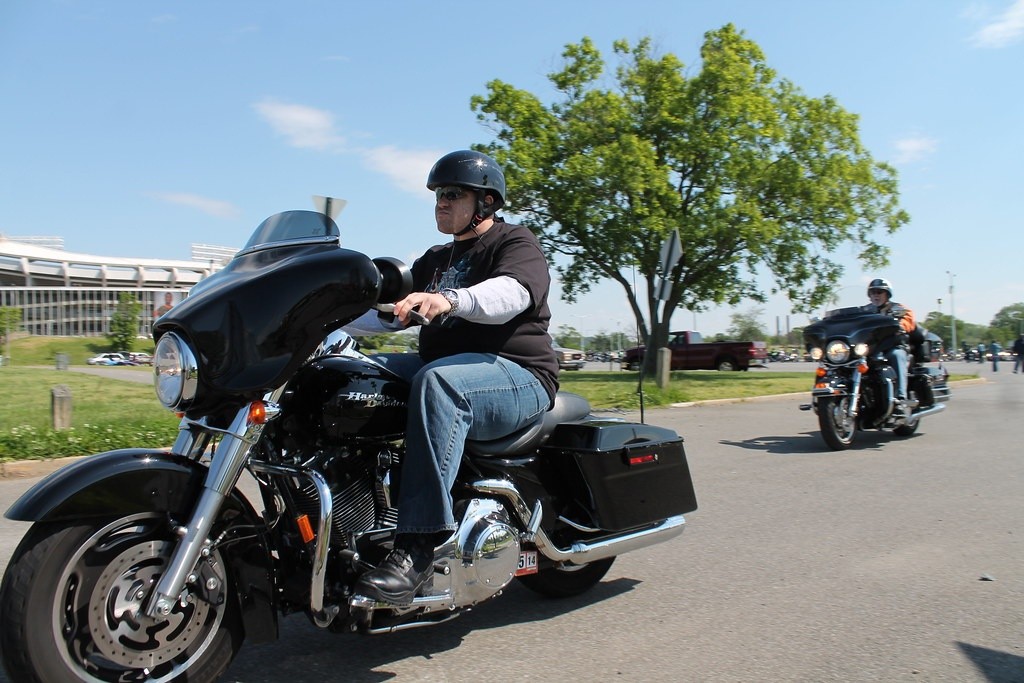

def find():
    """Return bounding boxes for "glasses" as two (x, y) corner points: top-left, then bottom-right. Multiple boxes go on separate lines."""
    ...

(870, 291), (883, 294)
(436, 189), (468, 201)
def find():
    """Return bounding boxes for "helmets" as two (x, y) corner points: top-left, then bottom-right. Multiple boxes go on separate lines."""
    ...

(426, 150), (505, 208)
(868, 279), (892, 298)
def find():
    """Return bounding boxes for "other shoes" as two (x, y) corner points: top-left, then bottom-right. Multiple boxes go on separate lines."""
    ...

(1013, 369), (1018, 373)
(894, 403), (906, 414)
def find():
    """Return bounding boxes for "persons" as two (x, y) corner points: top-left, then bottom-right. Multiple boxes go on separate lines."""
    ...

(158, 292), (174, 316)
(860, 279), (915, 406)
(342, 151), (561, 607)
(580, 330), (1024, 374)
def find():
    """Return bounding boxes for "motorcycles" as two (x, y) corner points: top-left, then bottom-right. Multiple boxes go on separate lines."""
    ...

(794, 308), (952, 451)
(584, 348), (621, 364)
(0, 207), (699, 682)
(761, 348), (799, 363)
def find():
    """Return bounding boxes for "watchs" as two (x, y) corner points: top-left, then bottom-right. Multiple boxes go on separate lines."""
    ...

(440, 290), (459, 317)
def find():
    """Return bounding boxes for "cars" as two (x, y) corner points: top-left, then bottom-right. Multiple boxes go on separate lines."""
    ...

(551, 339), (588, 372)
(86, 352), (154, 367)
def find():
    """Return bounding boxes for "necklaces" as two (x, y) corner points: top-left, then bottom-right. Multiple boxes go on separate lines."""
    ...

(448, 232), (486, 278)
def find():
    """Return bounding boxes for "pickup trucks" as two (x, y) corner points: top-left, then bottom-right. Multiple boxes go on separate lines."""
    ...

(620, 330), (768, 372)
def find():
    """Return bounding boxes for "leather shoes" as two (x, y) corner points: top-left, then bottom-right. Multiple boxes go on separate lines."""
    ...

(357, 548), (434, 605)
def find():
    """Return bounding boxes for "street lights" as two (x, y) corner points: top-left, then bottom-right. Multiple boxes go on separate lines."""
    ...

(945, 270), (959, 361)
